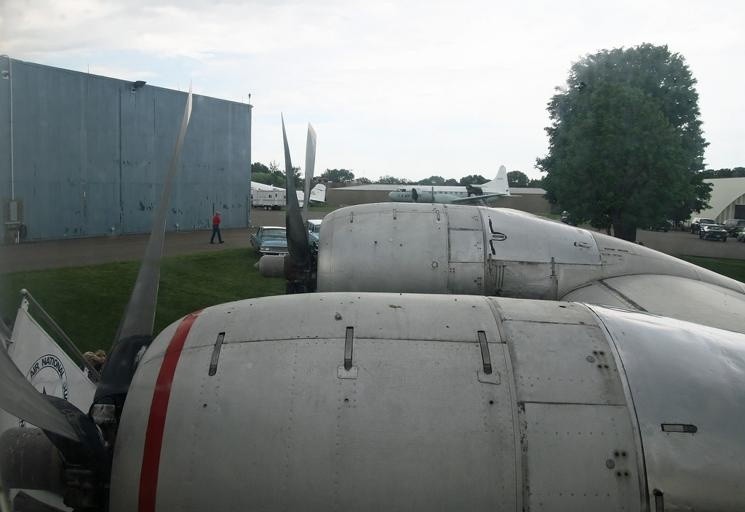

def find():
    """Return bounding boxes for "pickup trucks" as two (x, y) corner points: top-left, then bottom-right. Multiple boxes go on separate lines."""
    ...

(247, 225), (290, 257)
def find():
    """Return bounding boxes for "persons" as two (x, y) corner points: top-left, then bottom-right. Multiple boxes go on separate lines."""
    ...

(560, 211), (571, 225)
(210, 212), (225, 243)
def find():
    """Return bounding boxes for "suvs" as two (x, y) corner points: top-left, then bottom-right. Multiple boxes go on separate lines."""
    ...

(691, 218), (717, 234)
(307, 218), (322, 253)
(724, 219), (745, 236)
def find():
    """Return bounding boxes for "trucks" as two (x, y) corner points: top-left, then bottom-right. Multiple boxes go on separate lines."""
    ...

(251, 189), (286, 210)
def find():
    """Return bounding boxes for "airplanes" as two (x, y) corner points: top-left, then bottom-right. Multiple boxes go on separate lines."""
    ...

(0, 80), (745, 511)
(250, 180), (327, 208)
(388, 165), (522, 205)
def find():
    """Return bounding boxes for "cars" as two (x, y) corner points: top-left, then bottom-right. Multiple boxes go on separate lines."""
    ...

(560, 210), (572, 223)
(648, 220), (672, 232)
(679, 219), (691, 232)
(736, 231), (745, 242)
(698, 224), (728, 242)
